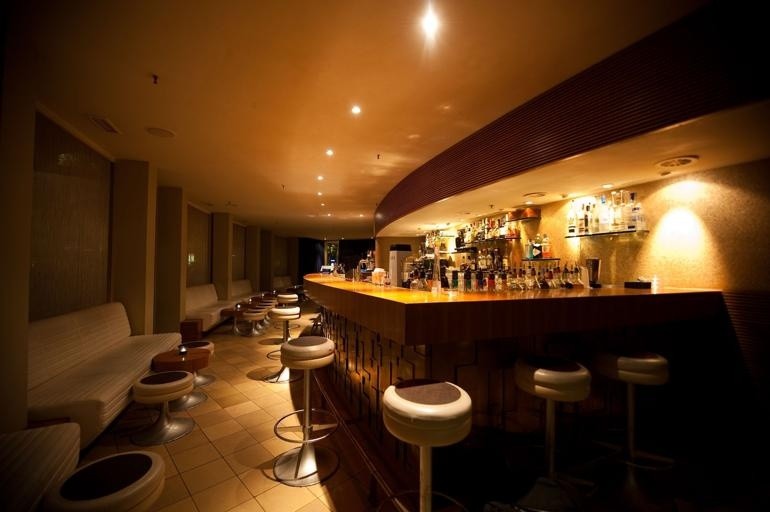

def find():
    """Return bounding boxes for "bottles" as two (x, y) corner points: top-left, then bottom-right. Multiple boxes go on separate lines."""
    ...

(453, 212), (554, 263)
(567, 190), (642, 236)
(409, 264), (581, 291)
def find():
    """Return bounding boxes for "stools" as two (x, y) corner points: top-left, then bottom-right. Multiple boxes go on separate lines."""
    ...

(178, 339), (217, 385)
(267, 307), (301, 361)
(274, 336), (342, 487)
(277, 294), (299, 343)
(51, 451), (166, 512)
(131, 370), (194, 445)
(513, 355), (593, 512)
(153, 350), (213, 409)
(597, 352), (675, 474)
(239, 296), (278, 337)
(378, 376), (476, 511)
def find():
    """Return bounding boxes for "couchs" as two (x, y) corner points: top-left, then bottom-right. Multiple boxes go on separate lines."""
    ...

(185, 283), (242, 331)
(0, 422), (80, 512)
(273, 276), (293, 291)
(27, 301), (183, 451)
(231, 278), (269, 303)
